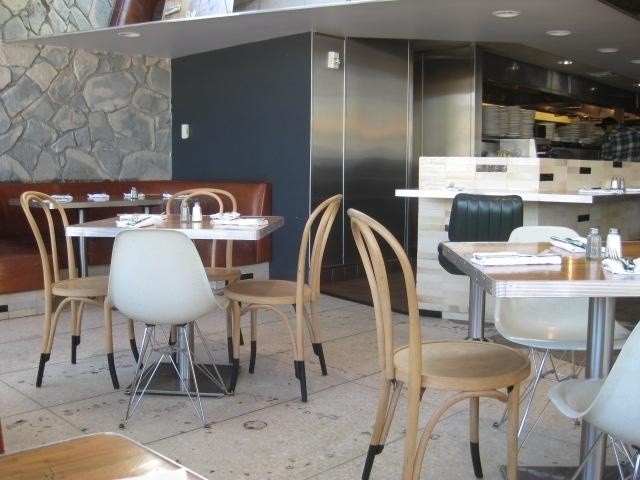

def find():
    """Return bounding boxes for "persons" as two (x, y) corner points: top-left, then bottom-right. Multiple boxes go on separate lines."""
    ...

(599, 116), (640, 162)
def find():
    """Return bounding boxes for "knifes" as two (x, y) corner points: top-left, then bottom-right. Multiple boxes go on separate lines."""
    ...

(550, 233), (587, 251)
(472, 248), (562, 262)
(132, 216), (151, 226)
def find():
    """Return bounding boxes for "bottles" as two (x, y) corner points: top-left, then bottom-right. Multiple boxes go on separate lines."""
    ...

(605, 227), (623, 259)
(179, 201), (190, 223)
(191, 200), (202, 221)
(610, 175), (626, 193)
(130, 186), (138, 199)
(585, 226), (603, 260)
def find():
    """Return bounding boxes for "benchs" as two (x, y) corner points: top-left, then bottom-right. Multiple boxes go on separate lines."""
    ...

(0, 180), (273, 320)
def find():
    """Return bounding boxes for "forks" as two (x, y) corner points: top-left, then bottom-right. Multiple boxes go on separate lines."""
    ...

(607, 247), (634, 273)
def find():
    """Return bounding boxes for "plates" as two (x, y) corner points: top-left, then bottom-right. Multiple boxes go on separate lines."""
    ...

(538, 120), (605, 145)
(481, 101), (537, 139)
